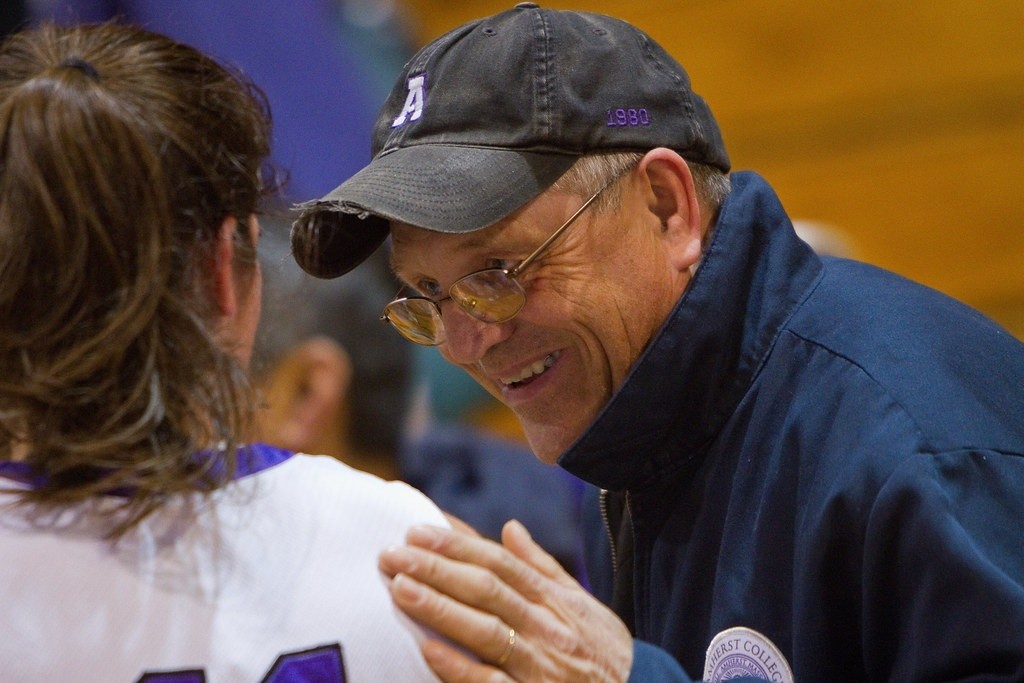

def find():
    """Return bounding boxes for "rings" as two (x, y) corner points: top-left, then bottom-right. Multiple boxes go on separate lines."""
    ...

(497, 624), (516, 670)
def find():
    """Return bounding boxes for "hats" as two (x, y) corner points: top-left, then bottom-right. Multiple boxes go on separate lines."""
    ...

(287, 2), (731, 281)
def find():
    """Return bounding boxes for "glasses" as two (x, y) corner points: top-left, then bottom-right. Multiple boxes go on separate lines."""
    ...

(379, 157), (643, 348)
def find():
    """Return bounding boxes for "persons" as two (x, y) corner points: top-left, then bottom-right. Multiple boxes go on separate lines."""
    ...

(0, 18), (502, 682)
(290, 2), (1022, 682)
